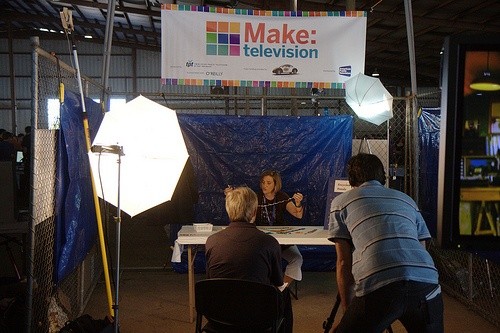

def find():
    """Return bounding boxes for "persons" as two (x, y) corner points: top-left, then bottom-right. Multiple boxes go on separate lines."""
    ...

(204, 186), (292, 333)
(327, 153), (445, 333)
(0, 126), (30, 190)
(224, 170), (303, 288)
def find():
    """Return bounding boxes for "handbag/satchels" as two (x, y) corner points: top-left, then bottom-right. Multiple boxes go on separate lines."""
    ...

(57, 314), (121, 333)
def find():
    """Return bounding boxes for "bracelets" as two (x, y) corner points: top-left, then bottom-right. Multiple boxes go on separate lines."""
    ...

(296, 204), (302, 207)
(296, 208), (302, 213)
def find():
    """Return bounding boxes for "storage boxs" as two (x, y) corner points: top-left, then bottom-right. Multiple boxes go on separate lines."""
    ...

(193, 223), (213, 232)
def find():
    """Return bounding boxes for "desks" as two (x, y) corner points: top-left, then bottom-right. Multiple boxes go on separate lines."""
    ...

(171, 223), (335, 324)
(459, 186), (500, 236)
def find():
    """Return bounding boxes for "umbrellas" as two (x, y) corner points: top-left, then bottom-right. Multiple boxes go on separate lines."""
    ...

(87, 94), (189, 217)
(345, 72), (394, 126)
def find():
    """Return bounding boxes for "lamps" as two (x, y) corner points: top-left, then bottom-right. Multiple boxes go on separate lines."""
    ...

(469, 48), (500, 91)
